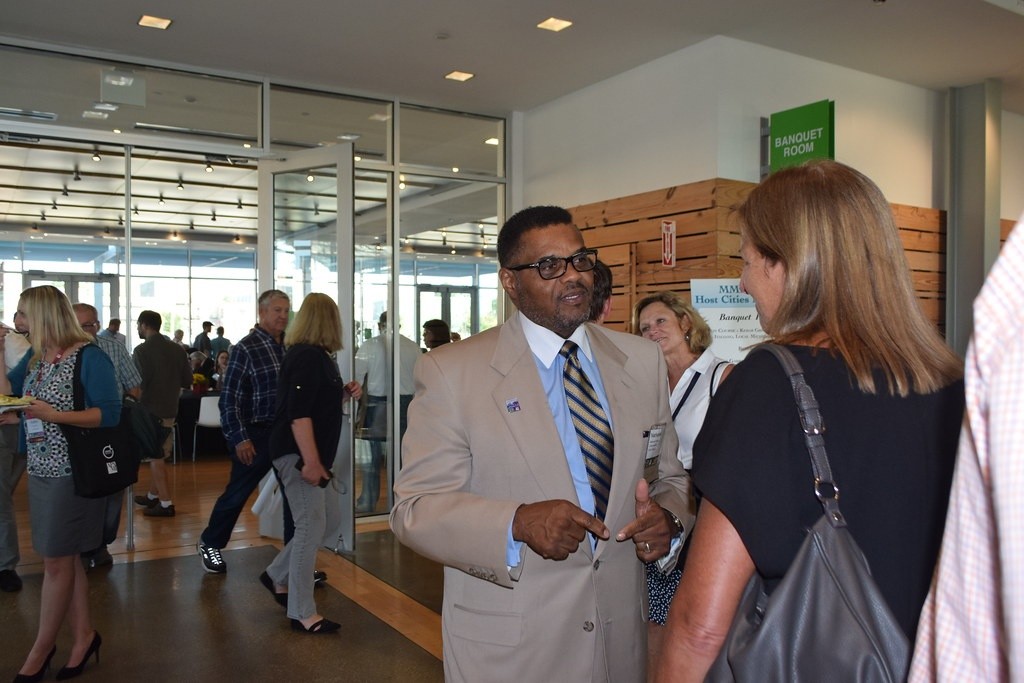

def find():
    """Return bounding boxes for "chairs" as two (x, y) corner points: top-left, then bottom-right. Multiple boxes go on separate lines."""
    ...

(162, 417), (182, 465)
(193, 396), (222, 463)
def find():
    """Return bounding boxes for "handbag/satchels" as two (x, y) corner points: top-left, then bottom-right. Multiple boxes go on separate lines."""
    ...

(69, 343), (140, 499)
(705, 343), (910, 683)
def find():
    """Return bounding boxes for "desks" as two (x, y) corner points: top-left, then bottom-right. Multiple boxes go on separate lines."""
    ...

(164, 390), (230, 461)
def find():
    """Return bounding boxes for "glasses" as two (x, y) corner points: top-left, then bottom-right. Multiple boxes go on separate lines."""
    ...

(81, 322), (97, 332)
(509, 249), (598, 280)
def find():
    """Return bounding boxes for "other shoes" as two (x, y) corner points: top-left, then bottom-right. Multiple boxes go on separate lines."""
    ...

(93, 548), (114, 565)
(260, 572), (288, 608)
(82, 558), (91, 572)
(0, 570), (22, 591)
(291, 618), (342, 634)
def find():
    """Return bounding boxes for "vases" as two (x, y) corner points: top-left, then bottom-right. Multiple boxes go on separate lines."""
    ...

(191, 383), (209, 395)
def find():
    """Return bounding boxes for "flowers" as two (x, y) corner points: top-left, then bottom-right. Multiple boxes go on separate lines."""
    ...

(193, 373), (209, 385)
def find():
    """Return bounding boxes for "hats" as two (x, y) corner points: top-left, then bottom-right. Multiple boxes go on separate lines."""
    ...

(203, 322), (213, 327)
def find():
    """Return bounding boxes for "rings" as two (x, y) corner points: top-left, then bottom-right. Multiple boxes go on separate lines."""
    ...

(645, 542), (649, 552)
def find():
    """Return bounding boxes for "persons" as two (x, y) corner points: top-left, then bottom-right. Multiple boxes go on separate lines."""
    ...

(0, 326), (32, 592)
(588, 260), (612, 326)
(632, 291), (737, 683)
(388, 204), (698, 683)
(423, 319), (451, 349)
(173, 321), (232, 388)
(355, 312), (423, 513)
(653, 160), (965, 683)
(196, 290), (326, 584)
(131, 310), (194, 517)
(72, 304), (142, 570)
(101, 318), (126, 344)
(905, 211), (1024, 683)
(0, 285), (122, 683)
(259, 292), (362, 634)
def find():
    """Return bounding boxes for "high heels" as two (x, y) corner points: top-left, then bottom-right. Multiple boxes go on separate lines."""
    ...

(56, 631), (102, 680)
(12, 644), (56, 683)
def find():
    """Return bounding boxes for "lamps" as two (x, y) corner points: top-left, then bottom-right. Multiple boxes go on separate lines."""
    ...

(314, 204), (320, 215)
(478, 224), (485, 239)
(173, 231), (177, 238)
(52, 201), (57, 209)
(41, 212), (46, 220)
(211, 213), (216, 220)
(91, 145), (101, 161)
(405, 236), (409, 244)
(134, 208), (138, 215)
(177, 177), (184, 190)
(441, 232), (448, 247)
(306, 175), (316, 184)
(237, 198), (243, 209)
(32, 223), (38, 229)
(62, 185), (68, 196)
(190, 222), (194, 229)
(399, 182), (406, 190)
(451, 249), (456, 255)
(375, 243), (381, 249)
(118, 217), (123, 225)
(205, 161), (214, 172)
(105, 227), (109, 234)
(158, 193), (165, 205)
(73, 170), (81, 181)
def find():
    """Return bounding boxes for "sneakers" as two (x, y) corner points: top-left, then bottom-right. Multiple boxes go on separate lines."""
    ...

(196, 538), (226, 573)
(143, 503), (176, 516)
(313, 571), (326, 583)
(134, 495), (159, 507)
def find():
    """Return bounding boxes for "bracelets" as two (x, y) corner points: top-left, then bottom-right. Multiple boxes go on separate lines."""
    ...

(670, 513), (680, 527)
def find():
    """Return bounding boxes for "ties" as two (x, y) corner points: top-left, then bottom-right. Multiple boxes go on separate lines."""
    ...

(559, 342), (615, 549)
